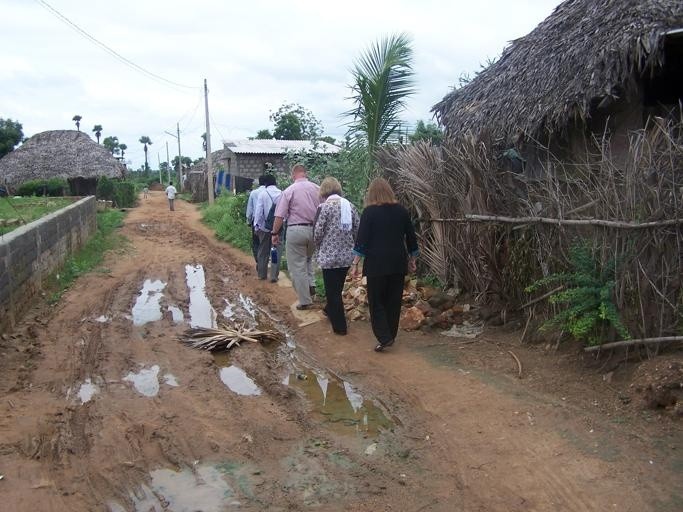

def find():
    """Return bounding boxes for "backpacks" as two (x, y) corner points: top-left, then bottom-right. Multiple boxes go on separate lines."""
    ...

(264, 190), (284, 230)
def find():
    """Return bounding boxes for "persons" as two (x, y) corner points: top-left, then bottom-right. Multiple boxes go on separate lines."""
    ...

(143, 185), (149, 200)
(244, 164), (419, 351)
(166, 181), (178, 212)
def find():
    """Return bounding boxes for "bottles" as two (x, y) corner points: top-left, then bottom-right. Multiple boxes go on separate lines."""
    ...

(269, 247), (279, 264)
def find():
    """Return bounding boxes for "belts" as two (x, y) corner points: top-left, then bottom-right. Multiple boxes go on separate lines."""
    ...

(289, 222), (313, 226)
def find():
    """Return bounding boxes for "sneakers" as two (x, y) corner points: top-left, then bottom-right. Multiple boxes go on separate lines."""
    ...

(296, 304), (312, 310)
(309, 286), (315, 296)
(322, 304), (328, 315)
(334, 329), (347, 335)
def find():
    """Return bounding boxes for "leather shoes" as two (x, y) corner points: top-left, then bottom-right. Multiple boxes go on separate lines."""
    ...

(375, 336), (395, 352)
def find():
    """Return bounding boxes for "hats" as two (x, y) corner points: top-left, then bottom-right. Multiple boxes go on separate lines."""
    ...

(265, 174), (276, 187)
